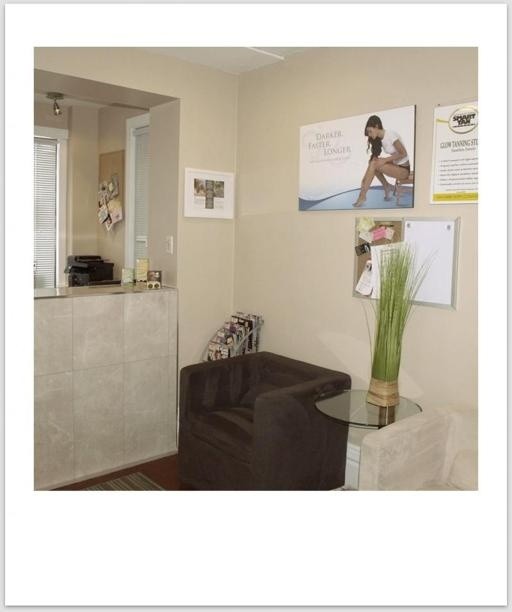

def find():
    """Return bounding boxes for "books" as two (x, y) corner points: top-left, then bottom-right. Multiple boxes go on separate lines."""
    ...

(206, 311), (264, 361)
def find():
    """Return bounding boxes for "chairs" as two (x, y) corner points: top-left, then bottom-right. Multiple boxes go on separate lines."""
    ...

(178, 352), (352, 490)
(357, 402), (478, 491)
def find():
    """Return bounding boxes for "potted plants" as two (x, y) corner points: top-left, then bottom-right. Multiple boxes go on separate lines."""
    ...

(358, 242), (439, 408)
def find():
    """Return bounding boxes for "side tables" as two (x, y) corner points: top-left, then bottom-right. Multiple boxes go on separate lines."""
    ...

(315, 388), (422, 429)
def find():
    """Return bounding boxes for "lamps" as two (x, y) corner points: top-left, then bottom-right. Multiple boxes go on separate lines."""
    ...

(45, 92), (64, 116)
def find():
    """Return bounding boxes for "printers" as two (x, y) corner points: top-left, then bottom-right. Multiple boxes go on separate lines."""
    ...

(65, 255), (114, 286)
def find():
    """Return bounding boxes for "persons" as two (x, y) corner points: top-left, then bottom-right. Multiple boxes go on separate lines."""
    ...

(352, 116), (411, 207)
